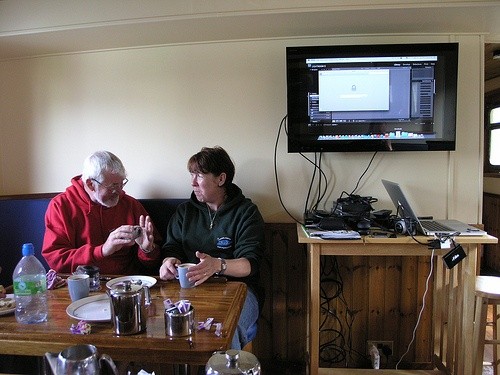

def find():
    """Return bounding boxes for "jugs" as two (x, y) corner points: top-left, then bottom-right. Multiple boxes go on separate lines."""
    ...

(45, 344), (119, 375)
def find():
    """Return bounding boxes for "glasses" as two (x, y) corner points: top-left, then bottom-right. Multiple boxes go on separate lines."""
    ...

(92, 178), (128, 190)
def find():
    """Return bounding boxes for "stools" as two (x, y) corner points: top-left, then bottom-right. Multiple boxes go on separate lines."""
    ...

(473, 275), (500, 375)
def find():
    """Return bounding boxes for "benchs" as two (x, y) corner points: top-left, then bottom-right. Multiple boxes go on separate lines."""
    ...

(0, 192), (187, 375)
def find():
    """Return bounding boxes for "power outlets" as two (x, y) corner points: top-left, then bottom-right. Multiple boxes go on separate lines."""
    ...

(367, 340), (394, 358)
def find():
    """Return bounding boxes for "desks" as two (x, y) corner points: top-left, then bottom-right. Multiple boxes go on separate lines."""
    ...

(295, 217), (498, 375)
(0, 273), (248, 375)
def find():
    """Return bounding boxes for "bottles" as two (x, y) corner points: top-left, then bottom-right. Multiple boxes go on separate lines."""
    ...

(12, 243), (48, 325)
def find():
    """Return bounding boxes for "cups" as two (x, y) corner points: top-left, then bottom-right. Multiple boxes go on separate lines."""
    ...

(164, 305), (195, 338)
(66, 274), (89, 302)
(106, 281), (146, 335)
(205, 350), (262, 375)
(174, 263), (196, 289)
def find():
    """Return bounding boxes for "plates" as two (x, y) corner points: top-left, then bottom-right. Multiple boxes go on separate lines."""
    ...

(0, 294), (15, 315)
(105, 275), (157, 291)
(65, 294), (111, 323)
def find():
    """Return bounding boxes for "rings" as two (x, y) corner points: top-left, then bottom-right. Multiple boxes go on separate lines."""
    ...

(204, 273), (207, 276)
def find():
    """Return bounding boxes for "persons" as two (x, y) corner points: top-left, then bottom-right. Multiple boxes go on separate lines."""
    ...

(160, 145), (263, 350)
(41, 150), (162, 276)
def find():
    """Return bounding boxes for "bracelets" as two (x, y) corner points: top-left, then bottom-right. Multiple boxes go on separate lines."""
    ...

(140, 244), (156, 253)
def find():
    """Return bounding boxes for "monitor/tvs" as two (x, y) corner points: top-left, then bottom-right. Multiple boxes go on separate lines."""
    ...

(286, 43), (459, 154)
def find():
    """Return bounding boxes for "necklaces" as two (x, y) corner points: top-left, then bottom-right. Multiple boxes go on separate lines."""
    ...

(207, 205), (217, 230)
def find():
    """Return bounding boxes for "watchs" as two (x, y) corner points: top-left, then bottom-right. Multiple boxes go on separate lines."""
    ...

(215, 257), (227, 275)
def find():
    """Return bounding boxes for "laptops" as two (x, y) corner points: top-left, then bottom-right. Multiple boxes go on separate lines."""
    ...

(382, 179), (488, 236)
